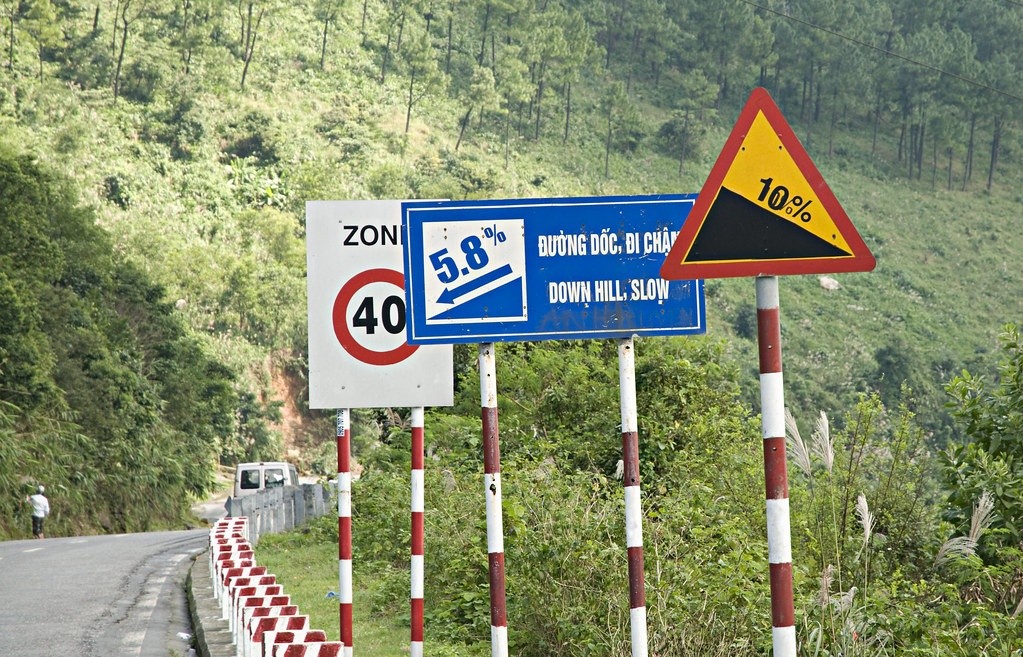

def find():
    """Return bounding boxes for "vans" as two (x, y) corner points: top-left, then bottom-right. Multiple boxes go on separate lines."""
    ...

(234, 462), (299, 501)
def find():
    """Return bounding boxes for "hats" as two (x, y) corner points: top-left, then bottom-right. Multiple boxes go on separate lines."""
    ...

(38, 485), (44, 493)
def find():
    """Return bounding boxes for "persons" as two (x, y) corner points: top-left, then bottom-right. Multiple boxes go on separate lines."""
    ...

(242, 471), (253, 484)
(26, 485), (50, 539)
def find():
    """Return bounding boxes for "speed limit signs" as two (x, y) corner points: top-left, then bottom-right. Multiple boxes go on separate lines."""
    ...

(332, 268), (420, 366)
